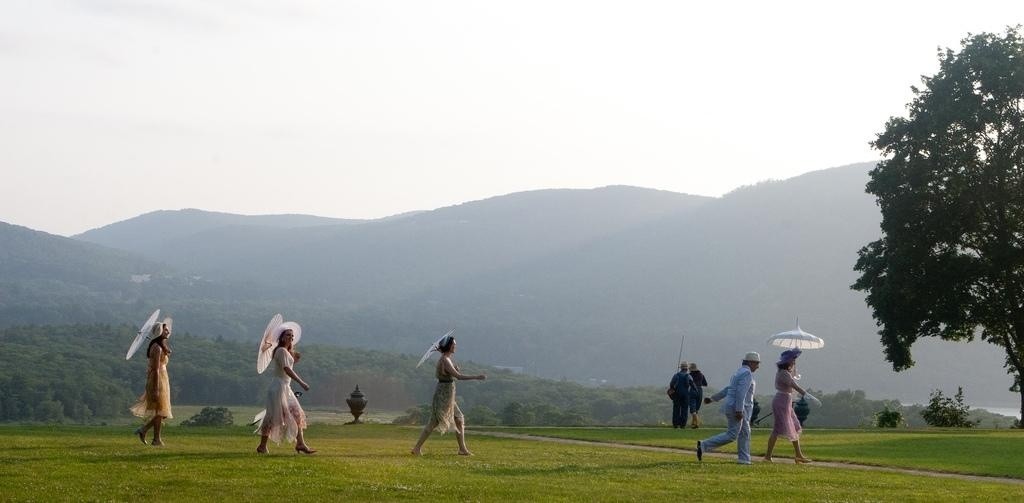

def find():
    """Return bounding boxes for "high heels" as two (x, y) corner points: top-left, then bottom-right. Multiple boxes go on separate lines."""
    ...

(152, 439), (164, 445)
(296, 446), (317, 454)
(764, 456), (772, 461)
(795, 457), (811, 463)
(134, 427), (147, 444)
(256, 445), (270, 454)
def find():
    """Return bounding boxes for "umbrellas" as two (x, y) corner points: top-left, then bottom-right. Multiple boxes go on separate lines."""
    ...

(256, 313), (283, 375)
(414, 327), (455, 369)
(768, 320), (824, 381)
(125, 308), (160, 361)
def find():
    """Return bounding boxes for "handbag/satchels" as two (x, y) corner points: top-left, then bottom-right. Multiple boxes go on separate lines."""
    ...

(667, 388), (676, 400)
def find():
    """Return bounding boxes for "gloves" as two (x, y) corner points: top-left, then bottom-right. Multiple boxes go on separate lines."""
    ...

(804, 393), (822, 408)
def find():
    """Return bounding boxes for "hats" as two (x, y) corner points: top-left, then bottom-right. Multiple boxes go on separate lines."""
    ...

(150, 317), (172, 341)
(743, 352), (760, 362)
(775, 347), (802, 365)
(274, 322), (302, 344)
(678, 361), (700, 371)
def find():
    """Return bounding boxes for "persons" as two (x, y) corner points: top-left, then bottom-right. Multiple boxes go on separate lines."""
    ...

(255, 321), (317, 455)
(669, 361), (699, 429)
(687, 362), (708, 429)
(763, 348), (821, 464)
(129, 312), (176, 447)
(695, 351), (762, 465)
(409, 335), (487, 457)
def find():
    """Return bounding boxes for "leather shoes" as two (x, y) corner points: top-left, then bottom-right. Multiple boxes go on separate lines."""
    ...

(697, 440), (702, 460)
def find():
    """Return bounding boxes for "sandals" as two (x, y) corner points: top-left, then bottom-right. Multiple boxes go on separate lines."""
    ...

(412, 449), (422, 454)
(459, 450), (474, 455)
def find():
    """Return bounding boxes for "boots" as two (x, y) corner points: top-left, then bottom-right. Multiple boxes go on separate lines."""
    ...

(690, 413), (702, 429)
